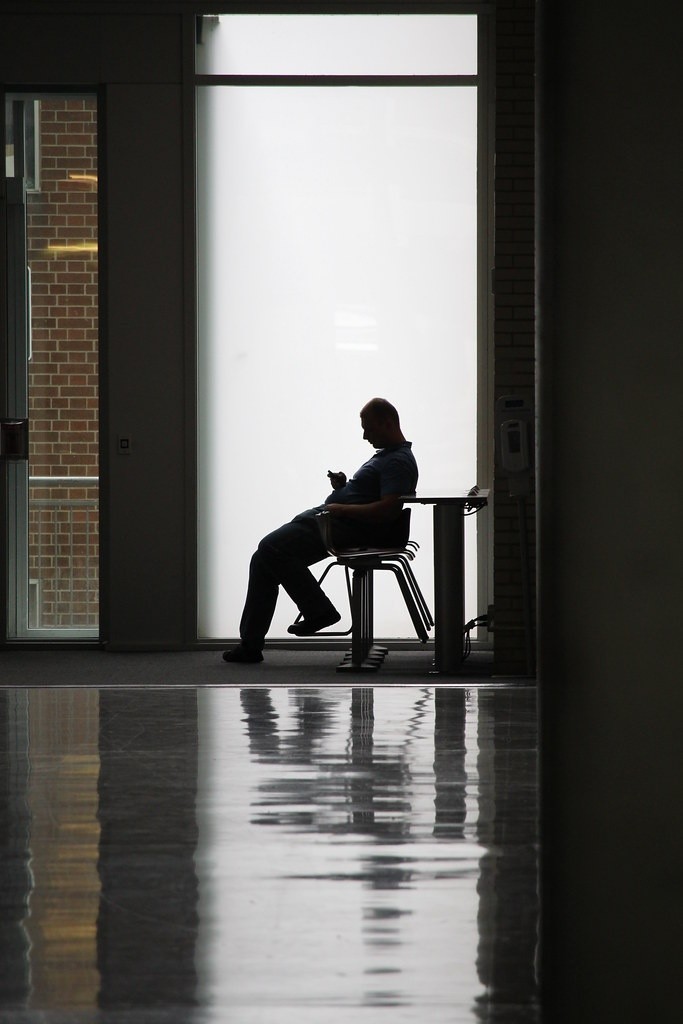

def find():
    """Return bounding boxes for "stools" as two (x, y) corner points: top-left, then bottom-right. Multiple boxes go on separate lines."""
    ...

(295, 508), (433, 674)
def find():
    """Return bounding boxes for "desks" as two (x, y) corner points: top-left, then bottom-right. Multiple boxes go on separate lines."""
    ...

(398, 495), (488, 675)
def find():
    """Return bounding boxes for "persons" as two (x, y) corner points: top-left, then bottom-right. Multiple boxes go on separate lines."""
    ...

(221, 396), (419, 664)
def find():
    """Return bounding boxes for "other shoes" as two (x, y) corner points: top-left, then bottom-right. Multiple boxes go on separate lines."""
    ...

(287, 610), (341, 634)
(222, 648), (263, 662)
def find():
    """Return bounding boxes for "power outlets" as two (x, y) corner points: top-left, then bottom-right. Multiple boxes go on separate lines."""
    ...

(487, 604), (496, 632)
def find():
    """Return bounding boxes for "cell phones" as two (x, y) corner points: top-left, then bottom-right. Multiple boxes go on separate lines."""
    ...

(328, 470), (334, 474)
